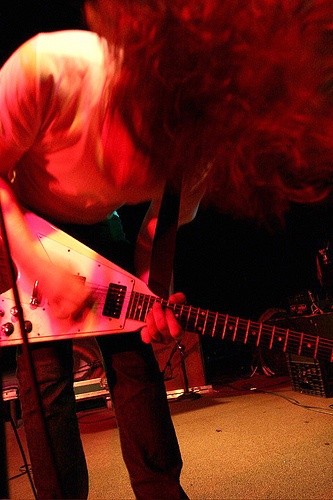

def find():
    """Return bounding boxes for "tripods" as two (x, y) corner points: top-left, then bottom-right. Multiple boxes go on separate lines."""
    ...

(308, 304), (325, 316)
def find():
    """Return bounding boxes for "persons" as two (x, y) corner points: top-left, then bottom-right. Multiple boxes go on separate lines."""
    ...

(0, 0), (333, 500)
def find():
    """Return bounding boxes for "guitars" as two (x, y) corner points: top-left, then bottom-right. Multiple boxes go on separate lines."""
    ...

(0, 205), (333, 361)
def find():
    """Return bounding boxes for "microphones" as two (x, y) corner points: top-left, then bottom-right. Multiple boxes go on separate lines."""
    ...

(166, 337), (181, 364)
(321, 249), (330, 264)
(308, 290), (316, 304)
